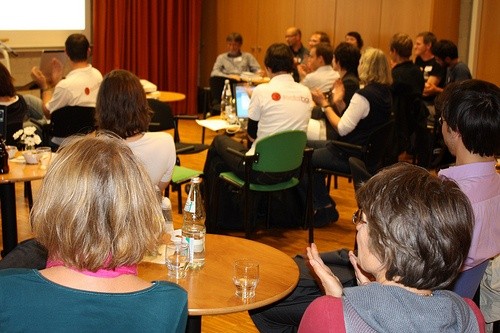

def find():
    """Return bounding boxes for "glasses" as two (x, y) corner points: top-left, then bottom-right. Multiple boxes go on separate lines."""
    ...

(436, 117), (447, 126)
(352, 210), (367, 225)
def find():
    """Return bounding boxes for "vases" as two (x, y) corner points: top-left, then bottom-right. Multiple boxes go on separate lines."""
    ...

(23, 140), (37, 151)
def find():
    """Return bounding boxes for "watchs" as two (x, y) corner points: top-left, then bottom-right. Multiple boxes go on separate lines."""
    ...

(321, 105), (332, 112)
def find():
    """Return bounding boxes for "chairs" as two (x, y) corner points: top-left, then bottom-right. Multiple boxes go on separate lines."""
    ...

(164, 165), (205, 215)
(42, 103), (100, 155)
(218, 128), (316, 248)
(145, 97), (211, 167)
(349, 156), (375, 249)
(201, 76), (256, 145)
(314, 118), (398, 198)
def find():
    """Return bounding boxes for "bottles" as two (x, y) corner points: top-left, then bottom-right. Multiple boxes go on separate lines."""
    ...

(220, 80), (233, 120)
(182, 177), (206, 269)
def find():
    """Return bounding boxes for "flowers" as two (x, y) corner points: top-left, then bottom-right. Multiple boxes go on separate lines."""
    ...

(12, 125), (42, 147)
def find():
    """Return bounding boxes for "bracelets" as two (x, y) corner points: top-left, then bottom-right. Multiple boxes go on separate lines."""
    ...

(40, 87), (49, 93)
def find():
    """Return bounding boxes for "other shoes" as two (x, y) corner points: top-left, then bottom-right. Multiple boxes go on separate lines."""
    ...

(313, 207), (339, 226)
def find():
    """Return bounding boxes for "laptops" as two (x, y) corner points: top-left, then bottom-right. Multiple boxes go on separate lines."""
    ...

(233, 83), (257, 129)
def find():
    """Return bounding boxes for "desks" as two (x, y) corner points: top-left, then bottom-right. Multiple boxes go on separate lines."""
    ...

(228, 74), (271, 87)
(205, 113), (251, 145)
(127, 233), (300, 333)
(0, 149), (58, 260)
(145, 91), (186, 132)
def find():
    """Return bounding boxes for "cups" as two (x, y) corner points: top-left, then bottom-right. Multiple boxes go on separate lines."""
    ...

(233, 258), (260, 300)
(36, 147), (52, 170)
(164, 242), (191, 279)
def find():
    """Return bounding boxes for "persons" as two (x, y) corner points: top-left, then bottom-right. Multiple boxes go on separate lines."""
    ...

(95, 67), (176, 204)
(299, 162), (488, 333)
(0, 129), (188, 333)
(185, 43), (313, 202)
(211, 32), (266, 82)
(0, 62), (44, 151)
(246, 78), (500, 333)
(285, 25), (474, 228)
(30, 34), (102, 152)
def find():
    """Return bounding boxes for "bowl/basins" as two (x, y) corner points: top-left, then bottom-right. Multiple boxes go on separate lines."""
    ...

(6, 146), (17, 158)
